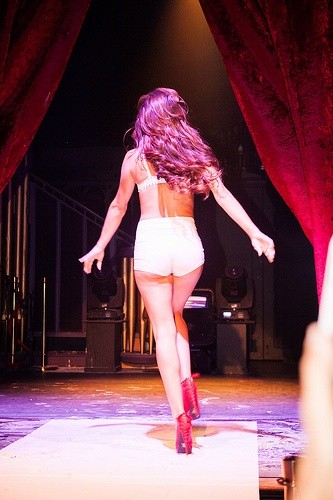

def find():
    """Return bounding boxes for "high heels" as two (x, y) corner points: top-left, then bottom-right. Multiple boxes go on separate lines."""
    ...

(175, 413), (193, 455)
(181, 378), (200, 421)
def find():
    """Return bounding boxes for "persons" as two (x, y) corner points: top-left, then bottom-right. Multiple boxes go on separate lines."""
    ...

(285, 233), (333, 500)
(78, 87), (277, 455)
